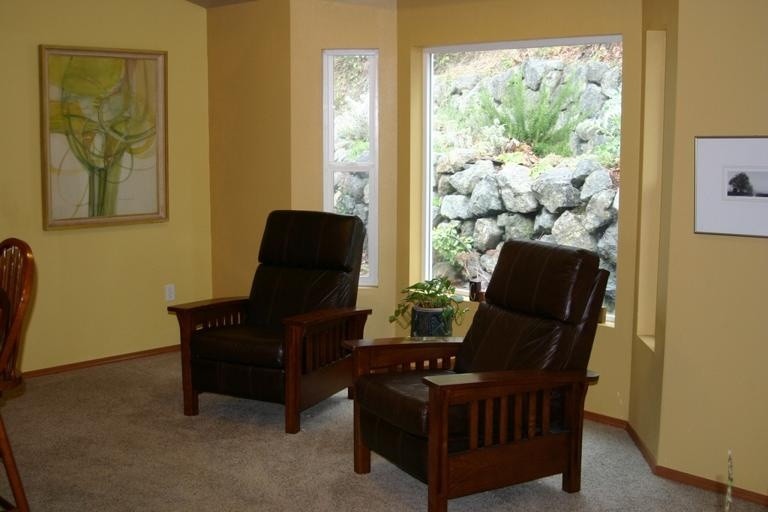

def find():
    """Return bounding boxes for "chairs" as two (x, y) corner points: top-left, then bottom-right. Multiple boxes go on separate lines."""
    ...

(0, 238), (36, 512)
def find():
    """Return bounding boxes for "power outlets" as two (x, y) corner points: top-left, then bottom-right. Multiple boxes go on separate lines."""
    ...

(165, 283), (175, 302)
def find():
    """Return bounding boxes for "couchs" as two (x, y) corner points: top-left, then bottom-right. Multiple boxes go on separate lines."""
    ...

(167, 209), (372, 434)
(340, 240), (608, 499)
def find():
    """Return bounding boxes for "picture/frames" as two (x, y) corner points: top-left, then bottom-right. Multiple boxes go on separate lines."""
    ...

(38, 42), (169, 230)
(694, 134), (764, 238)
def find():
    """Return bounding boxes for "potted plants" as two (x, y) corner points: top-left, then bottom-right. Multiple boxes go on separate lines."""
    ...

(386, 274), (469, 337)
